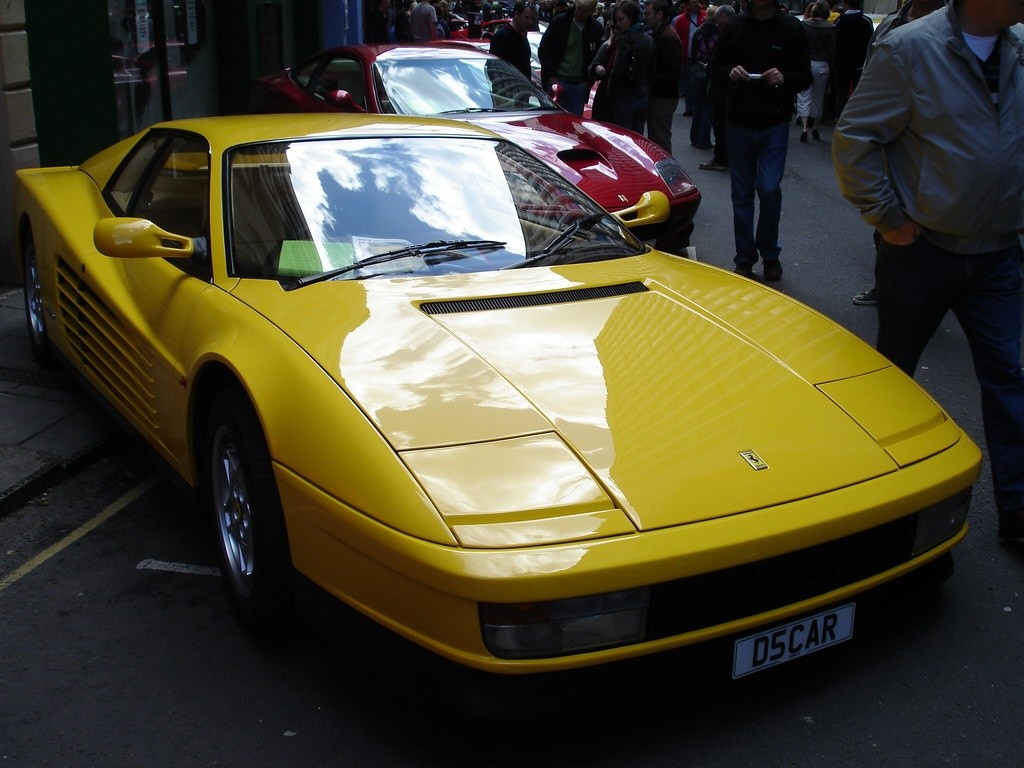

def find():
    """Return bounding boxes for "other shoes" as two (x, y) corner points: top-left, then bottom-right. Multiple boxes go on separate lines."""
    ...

(800, 132), (807, 142)
(808, 118), (814, 127)
(683, 111), (693, 116)
(853, 287), (878, 305)
(735, 264), (752, 277)
(697, 143), (715, 148)
(698, 159), (727, 170)
(812, 129), (819, 140)
(797, 117), (803, 126)
(998, 509), (1024, 544)
(763, 256), (783, 281)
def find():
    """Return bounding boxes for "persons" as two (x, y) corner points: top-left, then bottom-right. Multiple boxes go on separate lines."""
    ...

(362, 1), (1024, 557)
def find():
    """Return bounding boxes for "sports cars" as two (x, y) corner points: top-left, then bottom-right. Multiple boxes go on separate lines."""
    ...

(16, 111), (986, 693)
(451, 18), (601, 122)
(109, 40), (192, 127)
(248, 40), (702, 250)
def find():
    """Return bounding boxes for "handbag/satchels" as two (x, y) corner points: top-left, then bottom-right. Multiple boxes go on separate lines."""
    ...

(610, 35), (640, 90)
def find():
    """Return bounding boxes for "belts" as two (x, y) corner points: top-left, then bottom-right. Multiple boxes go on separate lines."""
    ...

(556, 74), (585, 83)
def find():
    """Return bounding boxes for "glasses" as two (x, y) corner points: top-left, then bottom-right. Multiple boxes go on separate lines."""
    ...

(611, 25), (619, 31)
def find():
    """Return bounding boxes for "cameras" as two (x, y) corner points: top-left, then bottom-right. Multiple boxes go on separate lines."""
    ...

(748, 74), (762, 79)
